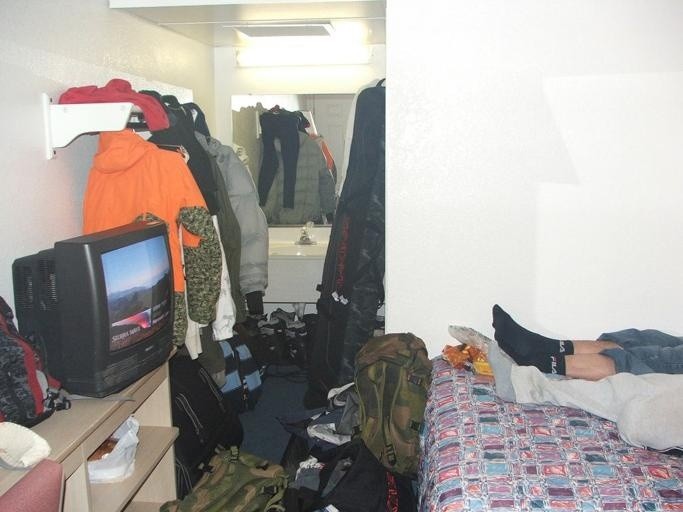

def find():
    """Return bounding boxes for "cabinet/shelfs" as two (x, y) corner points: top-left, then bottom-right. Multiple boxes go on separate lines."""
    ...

(0, 359), (180, 511)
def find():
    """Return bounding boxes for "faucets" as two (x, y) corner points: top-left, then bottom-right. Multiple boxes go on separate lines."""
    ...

(295, 226), (317, 244)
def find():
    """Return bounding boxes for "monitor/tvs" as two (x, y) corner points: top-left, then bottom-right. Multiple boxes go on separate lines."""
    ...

(12, 217), (174, 398)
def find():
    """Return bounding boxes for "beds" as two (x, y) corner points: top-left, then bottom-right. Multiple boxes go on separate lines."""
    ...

(427, 351), (683, 512)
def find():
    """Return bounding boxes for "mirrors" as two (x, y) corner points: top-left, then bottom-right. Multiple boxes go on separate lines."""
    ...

(231, 94), (358, 227)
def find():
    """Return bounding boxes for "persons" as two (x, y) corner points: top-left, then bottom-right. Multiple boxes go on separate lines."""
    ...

(485, 324), (682, 460)
(491, 303), (682, 381)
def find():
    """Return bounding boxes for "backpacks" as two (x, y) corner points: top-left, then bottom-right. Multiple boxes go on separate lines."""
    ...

(349, 326), (435, 484)
(157, 441), (292, 512)
(0, 294), (72, 431)
(171, 352), (244, 468)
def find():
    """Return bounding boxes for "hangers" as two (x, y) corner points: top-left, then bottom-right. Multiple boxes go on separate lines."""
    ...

(125, 120), (191, 164)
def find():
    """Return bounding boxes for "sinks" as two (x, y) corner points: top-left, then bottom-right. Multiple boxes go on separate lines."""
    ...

(271, 243), (328, 258)
